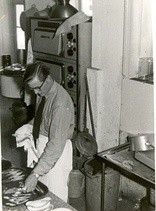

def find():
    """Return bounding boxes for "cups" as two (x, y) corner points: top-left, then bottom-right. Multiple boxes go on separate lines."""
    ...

(138, 56), (153, 82)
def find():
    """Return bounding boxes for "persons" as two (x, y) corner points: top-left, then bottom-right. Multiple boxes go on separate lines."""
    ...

(12, 60), (76, 205)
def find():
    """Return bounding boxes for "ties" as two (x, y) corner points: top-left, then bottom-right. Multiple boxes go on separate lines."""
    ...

(33, 96), (46, 138)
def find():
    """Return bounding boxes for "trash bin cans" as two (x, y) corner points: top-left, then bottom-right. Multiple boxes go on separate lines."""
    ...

(79, 156), (120, 211)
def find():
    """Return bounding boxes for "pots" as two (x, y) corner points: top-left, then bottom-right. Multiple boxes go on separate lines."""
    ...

(108, 134), (153, 155)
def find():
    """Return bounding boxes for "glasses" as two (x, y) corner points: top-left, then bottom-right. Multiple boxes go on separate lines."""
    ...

(29, 81), (45, 89)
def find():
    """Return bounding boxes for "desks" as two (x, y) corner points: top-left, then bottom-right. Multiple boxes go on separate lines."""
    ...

(94, 142), (155, 211)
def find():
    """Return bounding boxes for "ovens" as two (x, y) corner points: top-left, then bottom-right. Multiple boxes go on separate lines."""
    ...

(29, 17), (93, 158)
